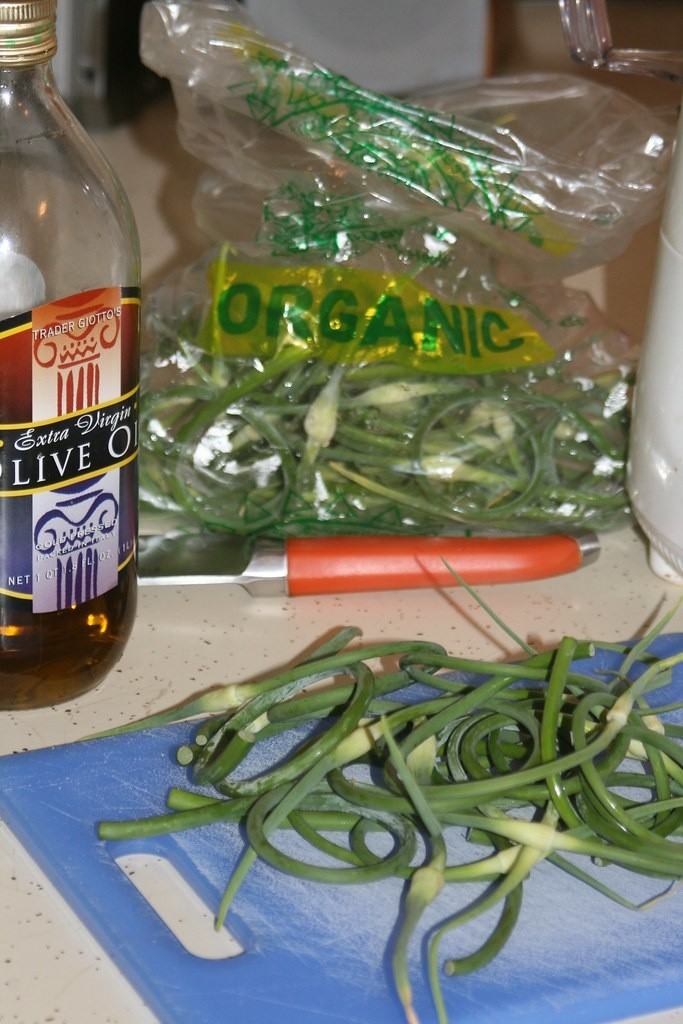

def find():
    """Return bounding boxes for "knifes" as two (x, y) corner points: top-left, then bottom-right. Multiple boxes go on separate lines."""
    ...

(139, 528), (601, 597)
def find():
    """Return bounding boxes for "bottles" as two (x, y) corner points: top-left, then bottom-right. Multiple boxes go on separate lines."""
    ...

(0, 0), (137, 716)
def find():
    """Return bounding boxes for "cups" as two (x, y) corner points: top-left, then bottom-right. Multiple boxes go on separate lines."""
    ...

(557, 0), (683, 583)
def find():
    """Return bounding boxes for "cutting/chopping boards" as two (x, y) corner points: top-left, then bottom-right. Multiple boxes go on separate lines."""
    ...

(0, 630), (683, 1024)
